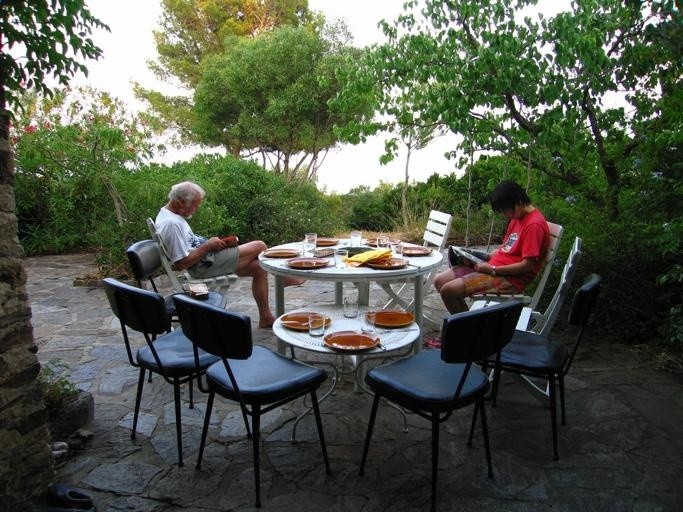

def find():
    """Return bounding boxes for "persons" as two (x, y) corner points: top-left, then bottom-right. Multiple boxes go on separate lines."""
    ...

(155, 180), (307, 328)
(433, 180), (551, 315)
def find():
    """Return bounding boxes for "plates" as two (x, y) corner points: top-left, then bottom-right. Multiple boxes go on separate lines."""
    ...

(281, 309), (415, 351)
(264, 241), (337, 269)
(366, 239), (433, 269)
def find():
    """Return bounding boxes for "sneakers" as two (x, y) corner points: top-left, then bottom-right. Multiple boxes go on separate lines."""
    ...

(48, 485), (92, 509)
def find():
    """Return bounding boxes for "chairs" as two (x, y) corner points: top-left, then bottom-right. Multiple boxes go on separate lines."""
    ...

(376, 209), (454, 332)
(359, 300), (525, 512)
(470, 222), (564, 311)
(127, 240), (228, 383)
(102, 278), (221, 468)
(171, 292), (332, 507)
(147, 219), (238, 294)
(467, 273), (602, 461)
(469, 236), (584, 409)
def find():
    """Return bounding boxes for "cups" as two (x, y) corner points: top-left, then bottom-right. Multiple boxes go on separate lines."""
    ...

(334, 250), (348, 270)
(351, 231), (362, 247)
(343, 287), (359, 318)
(305, 233), (317, 248)
(377, 237), (389, 248)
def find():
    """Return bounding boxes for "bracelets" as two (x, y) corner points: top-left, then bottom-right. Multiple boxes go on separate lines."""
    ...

(492, 265), (496, 276)
